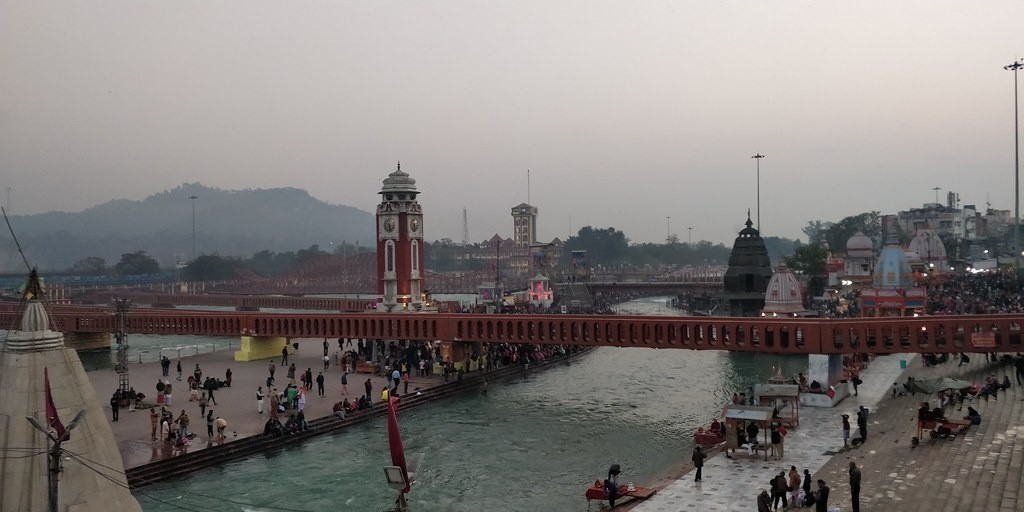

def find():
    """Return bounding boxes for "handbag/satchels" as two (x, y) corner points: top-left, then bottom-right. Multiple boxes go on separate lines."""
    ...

(794, 474), (800, 485)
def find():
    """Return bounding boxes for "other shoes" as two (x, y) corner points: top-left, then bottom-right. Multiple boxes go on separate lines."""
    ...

(784, 507), (788, 511)
(775, 509), (777, 512)
(699, 478), (701, 480)
(695, 478), (697, 481)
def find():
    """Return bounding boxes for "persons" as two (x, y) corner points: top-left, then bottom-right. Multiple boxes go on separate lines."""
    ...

(607, 464), (620, 489)
(674, 269), (1023, 458)
(691, 447), (704, 481)
(110, 290), (651, 449)
(757, 465), (828, 511)
(848, 461), (861, 512)
(604, 479), (616, 508)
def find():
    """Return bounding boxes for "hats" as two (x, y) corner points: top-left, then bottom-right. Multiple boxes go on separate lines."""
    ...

(762, 489), (767, 493)
